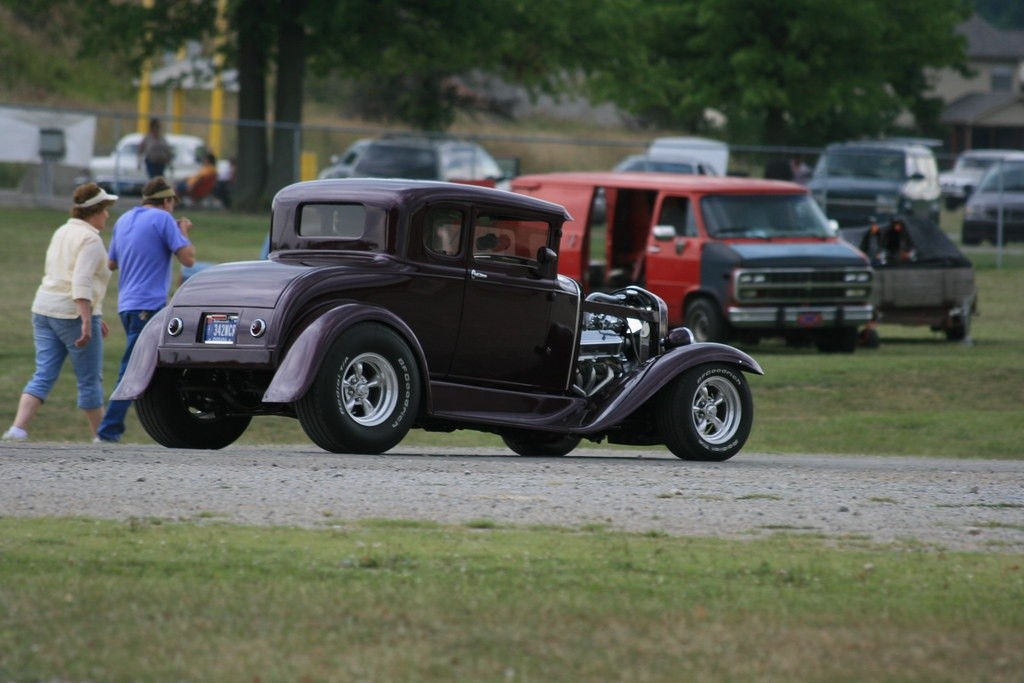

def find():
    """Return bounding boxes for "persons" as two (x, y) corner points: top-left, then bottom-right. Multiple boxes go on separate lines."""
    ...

(96, 176), (196, 442)
(764, 152), (813, 185)
(2, 182), (119, 442)
(860, 220), (918, 266)
(137, 119), (217, 209)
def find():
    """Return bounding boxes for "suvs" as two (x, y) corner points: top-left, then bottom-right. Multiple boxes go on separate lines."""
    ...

(814, 136), (947, 239)
(318, 128), (512, 200)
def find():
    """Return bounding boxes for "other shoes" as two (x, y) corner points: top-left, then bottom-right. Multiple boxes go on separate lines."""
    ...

(2, 430), (29, 443)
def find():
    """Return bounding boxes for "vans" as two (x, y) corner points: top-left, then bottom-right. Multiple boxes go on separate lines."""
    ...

(423, 170), (884, 351)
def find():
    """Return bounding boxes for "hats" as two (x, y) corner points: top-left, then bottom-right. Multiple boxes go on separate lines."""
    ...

(73, 186), (118, 208)
(143, 187), (180, 206)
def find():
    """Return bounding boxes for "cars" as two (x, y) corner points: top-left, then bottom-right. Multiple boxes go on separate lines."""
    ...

(942, 148), (1023, 210)
(109, 178), (765, 462)
(959, 153), (1024, 244)
(87, 132), (217, 203)
(799, 177), (980, 345)
(589, 138), (731, 218)
